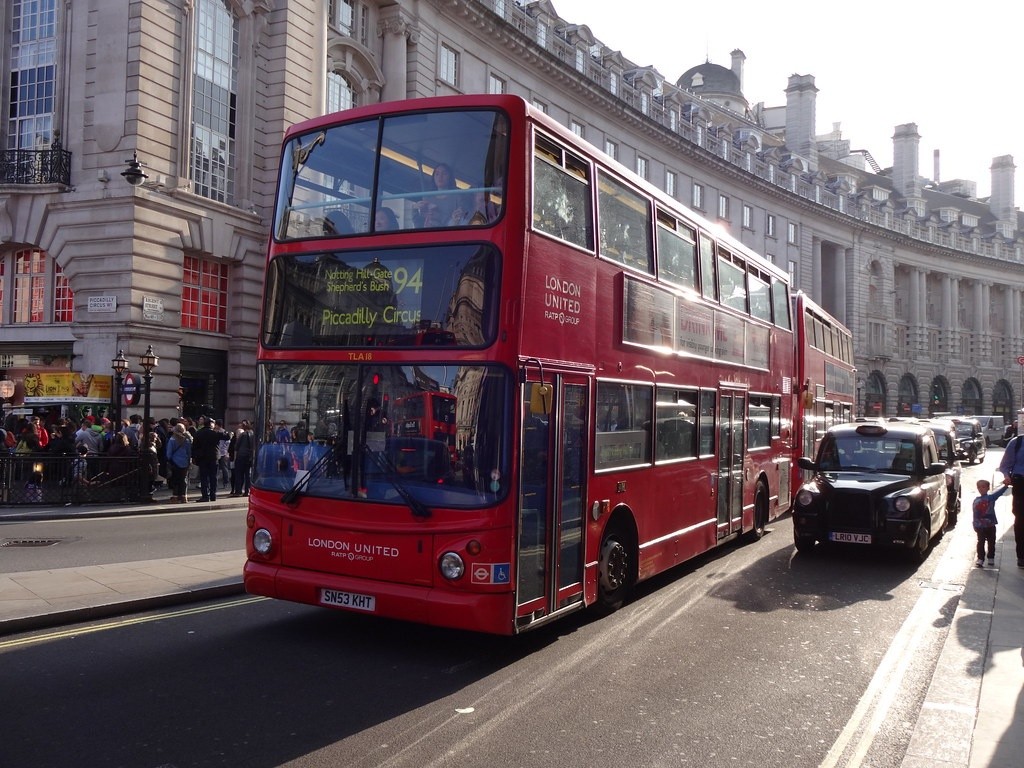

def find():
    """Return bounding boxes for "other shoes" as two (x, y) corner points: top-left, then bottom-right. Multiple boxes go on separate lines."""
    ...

(210, 495), (216, 501)
(988, 558), (994, 565)
(975, 558), (984, 565)
(196, 497), (209, 502)
(1017, 558), (1024, 569)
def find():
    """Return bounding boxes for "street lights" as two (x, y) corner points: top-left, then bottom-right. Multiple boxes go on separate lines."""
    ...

(140, 344), (158, 455)
(112, 348), (130, 435)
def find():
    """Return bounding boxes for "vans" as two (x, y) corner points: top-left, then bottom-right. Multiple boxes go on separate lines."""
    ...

(971, 415), (1006, 449)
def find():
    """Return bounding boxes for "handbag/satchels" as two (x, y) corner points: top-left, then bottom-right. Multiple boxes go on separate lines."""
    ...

(159, 462), (172, 478)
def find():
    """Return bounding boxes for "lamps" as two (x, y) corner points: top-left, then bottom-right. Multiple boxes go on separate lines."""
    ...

(120, 149), (149, 186)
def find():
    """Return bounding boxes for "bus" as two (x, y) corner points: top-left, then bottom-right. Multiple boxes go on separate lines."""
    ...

(242, 92), (857, 636)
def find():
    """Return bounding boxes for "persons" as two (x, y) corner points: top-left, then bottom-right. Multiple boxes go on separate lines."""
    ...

(840, 441), (870, 466)
(1003, 420), (1018, 444)
(322, 211), (356, 235)
(266, 419), (339, 478)
(972, 480), (1009, 567)
(999, 433), (1024, 569)
(0, 414), (255, 503)
(411, 163), (503, 227)
(370, 208), (400, 230)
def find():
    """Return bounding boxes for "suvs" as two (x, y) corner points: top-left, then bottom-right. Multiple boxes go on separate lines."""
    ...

(943, 415), (987, 466)
(894, 417), (969, 526)
(792, 421), (946, 562)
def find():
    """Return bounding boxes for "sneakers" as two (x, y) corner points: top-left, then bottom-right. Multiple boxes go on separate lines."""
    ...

(166, 496), (179, 503)
(180, 495), (188, 503)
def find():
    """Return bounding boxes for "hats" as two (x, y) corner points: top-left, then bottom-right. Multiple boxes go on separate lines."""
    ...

(216, 419), (223, 425)
(150, 417), (156, 425)
(204, 418), (215, 423)
(102, 417), (110, 423)
(87, 415), (95, 422)
